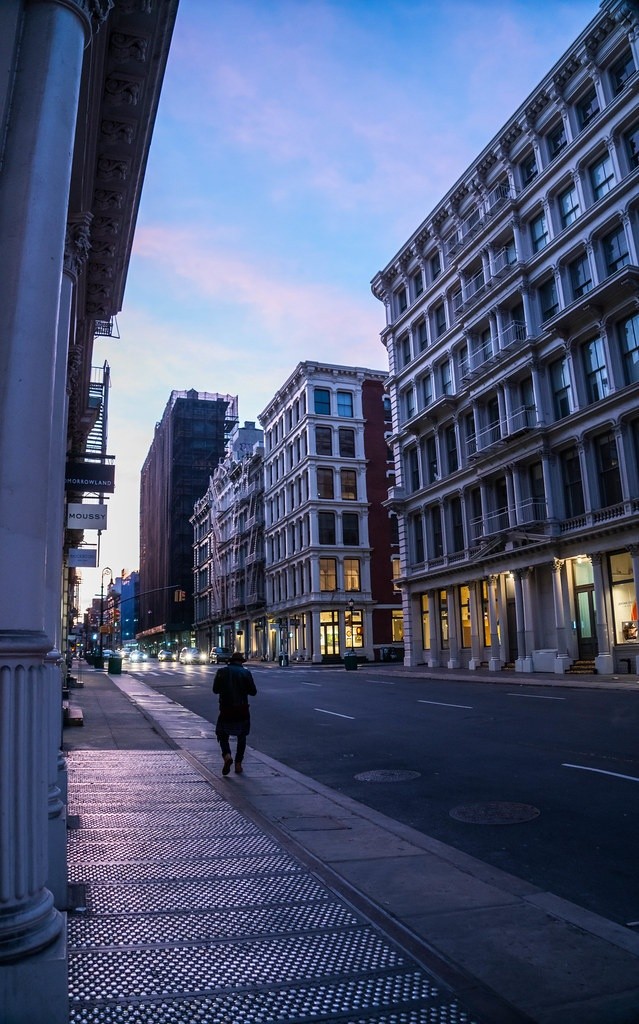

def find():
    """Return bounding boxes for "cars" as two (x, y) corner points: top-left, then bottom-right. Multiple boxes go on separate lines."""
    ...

(130, 650), (147, 663)
(158, 649), (177, 661)
(102, 649), (114, 660)
(120, 648), (131, 658)
(113, 650), (119, 655)
(179, 647), (206, 664)
(209, 647), (234, 664)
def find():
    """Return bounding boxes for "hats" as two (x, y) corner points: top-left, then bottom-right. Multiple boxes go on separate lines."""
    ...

(228, 653), (247, 663)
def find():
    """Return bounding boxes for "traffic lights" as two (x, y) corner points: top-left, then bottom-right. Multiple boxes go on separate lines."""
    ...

(92, 634), (97, 639)
(290, 633), (293, 638)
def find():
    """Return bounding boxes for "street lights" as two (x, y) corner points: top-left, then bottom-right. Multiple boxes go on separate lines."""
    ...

(175, 638), (178, 649)
(154, 641), (156, 657)
(99, 567), (116, 670)
(348, 597), (357, 653)
(85, 612), (93, 655)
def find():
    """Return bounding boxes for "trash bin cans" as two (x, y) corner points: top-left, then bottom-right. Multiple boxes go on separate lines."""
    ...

(278, 654), (289, 666)
(107, 653), (122, 675)
(343, 650), (359, 671)
(373, 646), (405, 664)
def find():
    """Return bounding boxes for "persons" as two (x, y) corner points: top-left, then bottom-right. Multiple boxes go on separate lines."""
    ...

(212, 652), (257, 776)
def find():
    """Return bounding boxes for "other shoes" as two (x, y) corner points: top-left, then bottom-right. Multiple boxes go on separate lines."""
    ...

(222, 754), (233, 775)
(234, 763), (244, 773)
